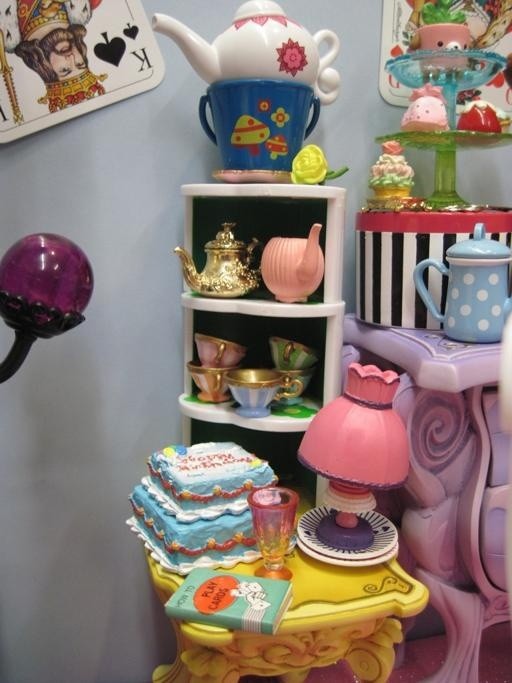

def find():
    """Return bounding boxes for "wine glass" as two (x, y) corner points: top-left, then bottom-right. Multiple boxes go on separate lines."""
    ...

(247, 487), (299, 580)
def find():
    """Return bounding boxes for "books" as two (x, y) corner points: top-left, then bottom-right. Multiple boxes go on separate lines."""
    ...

(164, 567), (293, 635)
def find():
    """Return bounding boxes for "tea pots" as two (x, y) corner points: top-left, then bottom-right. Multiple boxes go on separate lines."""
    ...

(260, 221), (325, 304)
(151, 1), (340, 111)
(414, 223), (510, 345)
(174, 220), (260, 296)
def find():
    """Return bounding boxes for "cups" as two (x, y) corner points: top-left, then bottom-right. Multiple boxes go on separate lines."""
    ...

(199, 82), (321, 176)
(405, 22), (472, 53)
(186, 334), (316, 418)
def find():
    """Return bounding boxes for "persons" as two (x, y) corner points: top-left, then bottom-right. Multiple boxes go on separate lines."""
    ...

(0, 0), (106, 114)
